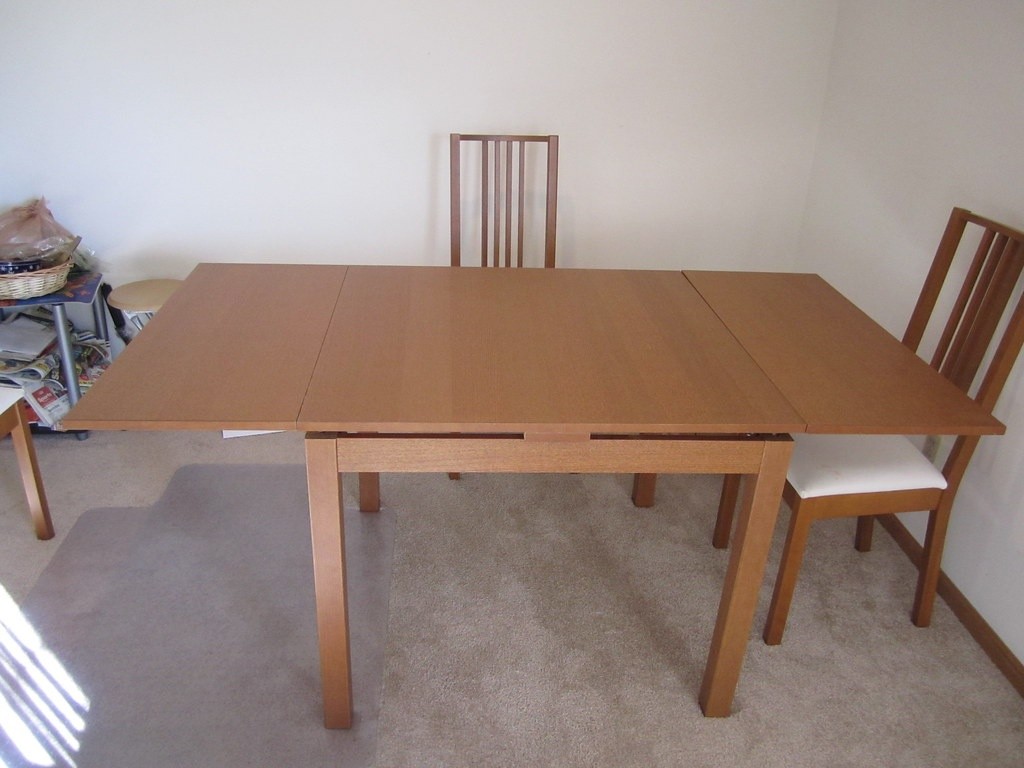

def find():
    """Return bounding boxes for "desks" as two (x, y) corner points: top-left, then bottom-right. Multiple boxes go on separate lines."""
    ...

(58, 262), (1005, 730)
(0, 386), (55, 540)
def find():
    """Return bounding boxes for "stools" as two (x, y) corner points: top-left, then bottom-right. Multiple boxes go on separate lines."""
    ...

(108, 279), (182, 331)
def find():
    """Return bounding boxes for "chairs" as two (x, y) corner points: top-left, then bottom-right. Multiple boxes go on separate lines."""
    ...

(763, 205), (1024, 647)
(449, 133), (559, 480)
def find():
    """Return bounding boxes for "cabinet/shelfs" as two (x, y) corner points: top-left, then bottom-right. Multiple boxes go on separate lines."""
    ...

(0, 271), (108, 441)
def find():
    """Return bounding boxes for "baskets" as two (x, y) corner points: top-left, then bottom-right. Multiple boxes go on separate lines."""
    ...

(0, 257), (74, 300)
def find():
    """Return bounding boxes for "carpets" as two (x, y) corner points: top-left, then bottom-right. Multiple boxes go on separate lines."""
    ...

(0, 461), (395, 768)
